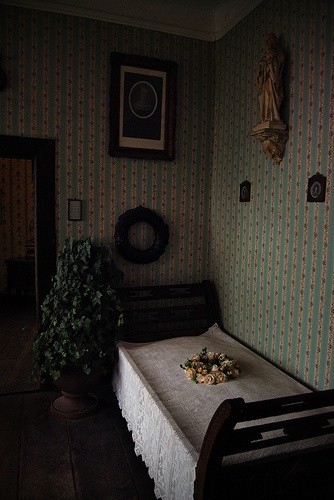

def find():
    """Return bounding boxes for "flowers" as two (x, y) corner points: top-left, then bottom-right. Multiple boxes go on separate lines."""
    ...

(180, 347), (239, 384)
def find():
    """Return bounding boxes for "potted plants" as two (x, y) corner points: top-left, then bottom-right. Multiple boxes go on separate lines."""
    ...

(31, 237), (126, 418)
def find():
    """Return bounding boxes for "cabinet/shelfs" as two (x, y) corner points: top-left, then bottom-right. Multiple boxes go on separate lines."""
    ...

(6, 255), (35, 299)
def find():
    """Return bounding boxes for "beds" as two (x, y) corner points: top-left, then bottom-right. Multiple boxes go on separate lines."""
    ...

(109, 281), (334, 499)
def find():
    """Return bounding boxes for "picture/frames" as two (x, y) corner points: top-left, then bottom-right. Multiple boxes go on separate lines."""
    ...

(68, 199), (82, 221)
(109, 53), (177, 160)
